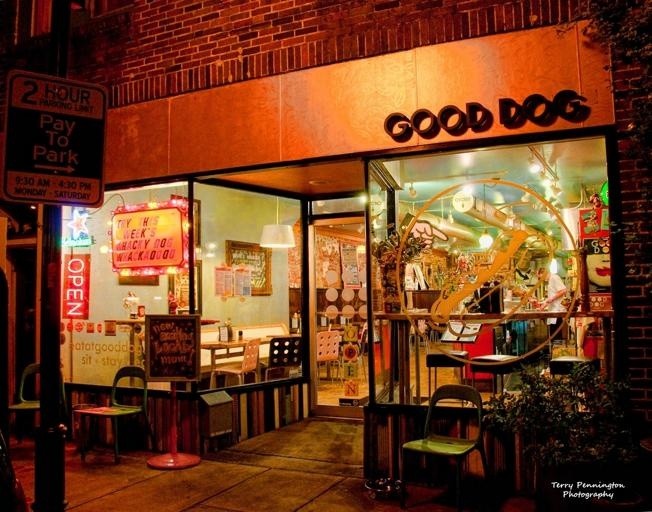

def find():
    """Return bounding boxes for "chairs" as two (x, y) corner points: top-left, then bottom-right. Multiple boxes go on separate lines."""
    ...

(73, 366), (156, 466)
(401, 385), (493, 492)
(9, 363), (69, 442)
(200, 309), (429, 389)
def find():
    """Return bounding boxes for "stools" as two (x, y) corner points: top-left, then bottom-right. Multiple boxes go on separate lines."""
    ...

(549, 357), (601, 380)
(425, 352), (469, 406)
(471, 355), (520, 403)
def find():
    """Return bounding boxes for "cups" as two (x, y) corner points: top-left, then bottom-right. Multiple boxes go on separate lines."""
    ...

(503, 298), (510, 313)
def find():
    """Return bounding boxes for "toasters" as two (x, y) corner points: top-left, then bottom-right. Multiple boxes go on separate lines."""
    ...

(239, 330), (243, 341)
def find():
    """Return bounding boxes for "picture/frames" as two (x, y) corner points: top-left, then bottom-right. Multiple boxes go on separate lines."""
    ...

(168, 260), (203, 317)
(225, 238), (272, 296)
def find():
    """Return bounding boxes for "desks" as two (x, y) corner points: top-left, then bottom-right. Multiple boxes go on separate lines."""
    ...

(369, 311), (613, 406)
(105, 319), (220, 388)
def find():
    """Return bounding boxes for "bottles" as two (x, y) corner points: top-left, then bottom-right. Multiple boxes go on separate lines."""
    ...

(226, 317), (233, 341)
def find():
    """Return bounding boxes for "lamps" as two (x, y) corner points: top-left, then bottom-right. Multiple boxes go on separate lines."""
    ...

(259, 195), (296, 249)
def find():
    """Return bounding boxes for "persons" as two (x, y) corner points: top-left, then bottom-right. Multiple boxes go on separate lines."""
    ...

(536, 268), (567, 340)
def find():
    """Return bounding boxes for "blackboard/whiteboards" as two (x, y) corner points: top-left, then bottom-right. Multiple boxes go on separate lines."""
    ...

(145, 314), (200, 382)
